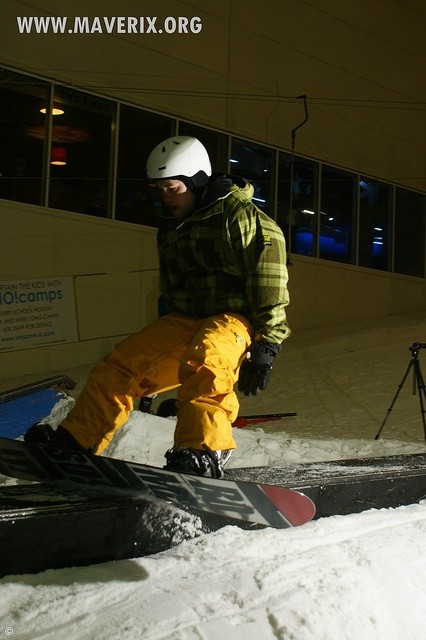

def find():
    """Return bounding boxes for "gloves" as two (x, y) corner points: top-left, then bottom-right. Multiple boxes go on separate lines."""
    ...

(237, 339), (280, 398)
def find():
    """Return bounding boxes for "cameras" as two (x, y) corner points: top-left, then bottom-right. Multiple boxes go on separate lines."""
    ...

(408, 342), (426, 358)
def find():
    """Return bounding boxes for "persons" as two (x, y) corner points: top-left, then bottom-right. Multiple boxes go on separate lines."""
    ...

(24, 135), (292, 479)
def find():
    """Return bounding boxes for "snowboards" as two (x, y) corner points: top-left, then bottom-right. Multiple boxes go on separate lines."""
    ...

(0, 437), (316, 528)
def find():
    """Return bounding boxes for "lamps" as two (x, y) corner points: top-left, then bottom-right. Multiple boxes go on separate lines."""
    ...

(40, 101), (64, 115)
(49, 146), (68, 166)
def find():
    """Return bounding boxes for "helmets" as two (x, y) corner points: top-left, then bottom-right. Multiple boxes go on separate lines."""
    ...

(146, 134), (212, 188)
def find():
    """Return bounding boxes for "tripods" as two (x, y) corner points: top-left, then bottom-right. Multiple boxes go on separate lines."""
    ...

(375, 358), (426, 443)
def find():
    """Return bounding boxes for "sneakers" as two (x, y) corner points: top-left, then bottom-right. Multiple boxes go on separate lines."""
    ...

(24, 422), (93, 456)
(166, 446), (225, 480)
